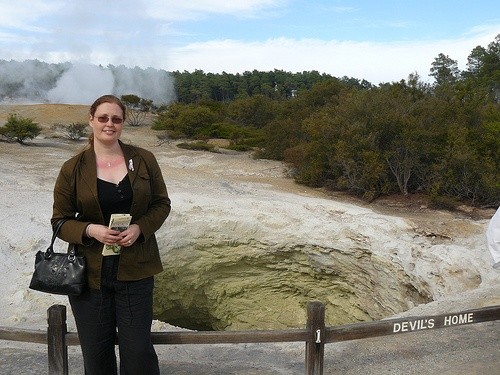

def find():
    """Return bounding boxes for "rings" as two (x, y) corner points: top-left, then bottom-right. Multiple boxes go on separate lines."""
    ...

(128, 239), (132, 244)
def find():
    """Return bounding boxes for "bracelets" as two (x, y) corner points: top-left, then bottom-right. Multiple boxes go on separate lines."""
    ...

(85, 224), (91, 239)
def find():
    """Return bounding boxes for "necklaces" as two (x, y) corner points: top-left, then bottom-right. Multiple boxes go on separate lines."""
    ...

(93, 151), (121, 167)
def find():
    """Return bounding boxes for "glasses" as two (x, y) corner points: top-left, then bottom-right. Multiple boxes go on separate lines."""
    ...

(93, 114), (124, 124)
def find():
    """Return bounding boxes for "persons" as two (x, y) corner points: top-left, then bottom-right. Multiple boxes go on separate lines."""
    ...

(51, 96), (171, 375)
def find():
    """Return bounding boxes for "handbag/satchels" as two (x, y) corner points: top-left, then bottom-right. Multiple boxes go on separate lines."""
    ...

(28, 218), (89, 298)
